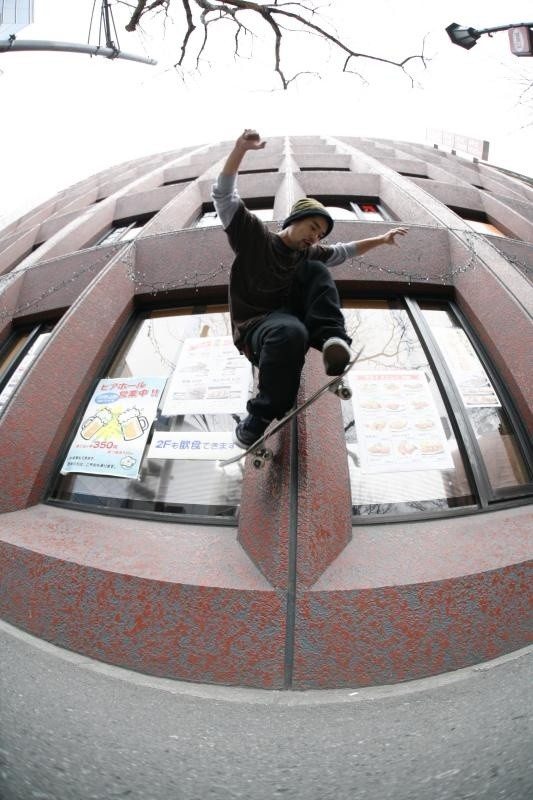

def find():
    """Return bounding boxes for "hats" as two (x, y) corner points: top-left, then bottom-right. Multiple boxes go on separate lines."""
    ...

(283, 198), (334, 241)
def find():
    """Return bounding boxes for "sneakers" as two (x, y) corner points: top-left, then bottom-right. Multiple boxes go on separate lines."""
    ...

(234, 413), (273, 449)
(322, 336), (351, 376)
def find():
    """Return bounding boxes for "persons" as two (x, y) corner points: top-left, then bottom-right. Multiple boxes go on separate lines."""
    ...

(210, 129), (409, 450)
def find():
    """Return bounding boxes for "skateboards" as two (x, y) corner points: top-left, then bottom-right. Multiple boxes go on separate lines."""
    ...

(218, 344), (367, 469)
